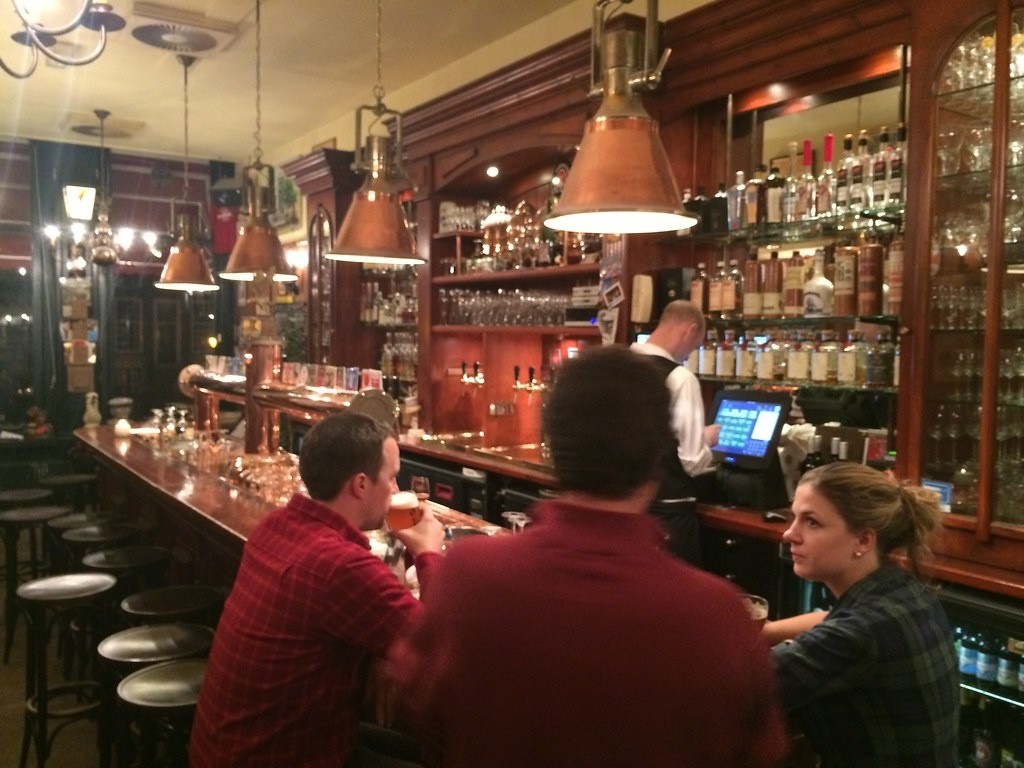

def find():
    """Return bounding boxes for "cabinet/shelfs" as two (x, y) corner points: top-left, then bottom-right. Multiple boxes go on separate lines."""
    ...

(891, 0), (1024, 573)
(423, 91), (618, 447)
(928, 585), (1023, 768)
(355, 263), (420, 433)
(304, 186), (388, 370)
(628, 38), (911, 523)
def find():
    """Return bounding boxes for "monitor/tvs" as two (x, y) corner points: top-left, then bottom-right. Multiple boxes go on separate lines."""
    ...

(705, 390), (790, 473)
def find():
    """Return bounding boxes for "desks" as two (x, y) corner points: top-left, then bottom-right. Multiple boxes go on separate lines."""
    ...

(72, 419), (512, 598)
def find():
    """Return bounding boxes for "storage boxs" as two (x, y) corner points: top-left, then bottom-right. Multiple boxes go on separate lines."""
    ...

(62, 291), (97, 392)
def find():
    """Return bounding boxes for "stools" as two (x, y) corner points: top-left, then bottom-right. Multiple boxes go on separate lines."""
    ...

(81, 544), (175, 587)
(0, 486), (54, 583)
(0, 503), (71, 668)
(15, 571), (127, 767)
(118, 655), (210, 768)
(37, 471), (100, 511)
(116, 583), (223, 622)
(45, 508), (124, 581)
(94, 620), (217, 768)
(59, 522), (147, 680)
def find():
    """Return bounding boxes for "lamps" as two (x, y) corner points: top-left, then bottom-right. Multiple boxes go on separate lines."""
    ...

(152, 53), (221, 294)
(0, 0), (126, 79)
(541, 0), (704, 236)
(215, 0), (301, 284)
(62, 173), (98, 240)
(320, 0), (428, 267)
(87, 109), (121, 264)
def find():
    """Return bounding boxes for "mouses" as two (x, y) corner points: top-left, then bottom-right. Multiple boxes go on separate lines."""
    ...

(763, 512), (786, 522)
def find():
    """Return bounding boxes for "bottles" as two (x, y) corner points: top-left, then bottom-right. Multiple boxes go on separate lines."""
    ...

(681, 122), (906, 387)
(799, 435), (849, 474)
(362, 282), (418, 380)
(949, 621), (1024, 768)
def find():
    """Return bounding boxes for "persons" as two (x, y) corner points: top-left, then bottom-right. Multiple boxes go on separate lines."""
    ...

(371, 345), (788, 768)
(626, 299), (722, 561)
(763, 462), (961, 768)
(187, 411), (446, 768)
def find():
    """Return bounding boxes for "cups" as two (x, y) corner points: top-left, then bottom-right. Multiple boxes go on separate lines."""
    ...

(436, 287), (571, 327)
(152, 406), (308, 506)
(736, 593), (770, 632)
(412, 477), (431, 502)
(387, 487), (421, 532)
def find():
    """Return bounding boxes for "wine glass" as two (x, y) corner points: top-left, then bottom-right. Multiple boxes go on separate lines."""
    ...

(925, 15), (1024, 525)
(501, 510), (530, 533)
